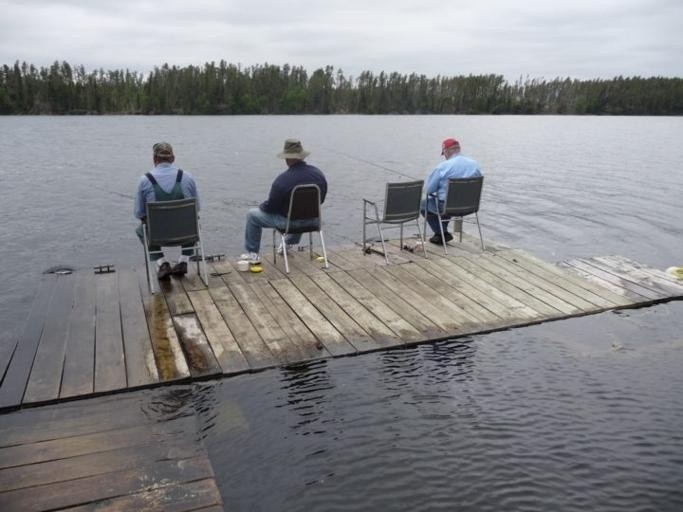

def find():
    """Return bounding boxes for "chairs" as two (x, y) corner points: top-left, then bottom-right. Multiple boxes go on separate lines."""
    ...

(418, 174), (488, 256)
(137, 195), (209, 296)
(269, 181), (329, 275)
(359, 178), (428, 266)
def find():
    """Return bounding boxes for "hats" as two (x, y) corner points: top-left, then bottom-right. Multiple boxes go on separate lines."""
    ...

(275, 137), (313, 162)
(150, 141), (174, 159)
(438, 136), (461, 156)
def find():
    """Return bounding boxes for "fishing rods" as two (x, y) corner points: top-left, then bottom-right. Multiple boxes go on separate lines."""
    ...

(332, 232), (389, 258)
(320, 146), (418, 180)
(94, 185), (135, 201)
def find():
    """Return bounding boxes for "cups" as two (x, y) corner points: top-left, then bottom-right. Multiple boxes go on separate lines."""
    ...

(249, 248), (263, 273)
(452, 217), (460, 233)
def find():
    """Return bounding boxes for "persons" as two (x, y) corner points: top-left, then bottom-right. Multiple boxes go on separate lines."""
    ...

(420, 138), (481, 243)
(240, 138), (328, 265)
(133, 141), (200, 279)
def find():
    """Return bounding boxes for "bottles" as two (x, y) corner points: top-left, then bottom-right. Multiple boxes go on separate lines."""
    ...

(237, 255), (248, 271)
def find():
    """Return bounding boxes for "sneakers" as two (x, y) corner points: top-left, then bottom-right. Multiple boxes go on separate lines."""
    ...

(429, 233), (442, 245)
(155, 261), (172, 281)
(276, 240), (298, 256)
(171, 260), (188, 280)
(239, 251), (263, 266)
(441, 231), (454, 243)
